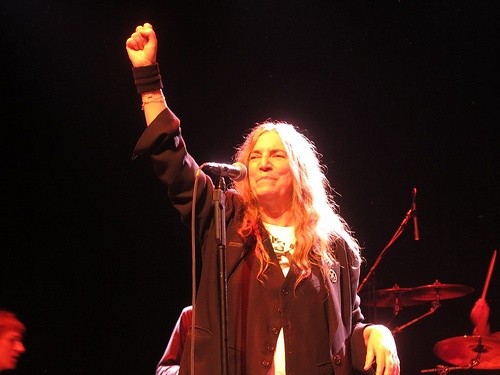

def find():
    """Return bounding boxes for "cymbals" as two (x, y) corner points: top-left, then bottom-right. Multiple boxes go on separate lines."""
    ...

(433, 335), (500, 371)
(357, 285), (417, 307)
(402, 280), (478, 302)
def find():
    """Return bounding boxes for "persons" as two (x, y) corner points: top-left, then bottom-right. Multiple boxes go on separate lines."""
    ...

(1, 312), (25, 368)
(470, 299), (500, 340)
(127, 23), (401, 375)
(156, 305), (194, 375)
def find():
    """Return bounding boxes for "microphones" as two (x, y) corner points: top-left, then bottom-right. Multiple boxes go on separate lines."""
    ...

(204, 162), (247, 182)
(412, 191), (419, 240)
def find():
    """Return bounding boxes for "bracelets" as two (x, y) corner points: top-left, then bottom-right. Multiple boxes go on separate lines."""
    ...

(142, 96), (166, 111)
(131, 63), (163, 94)
